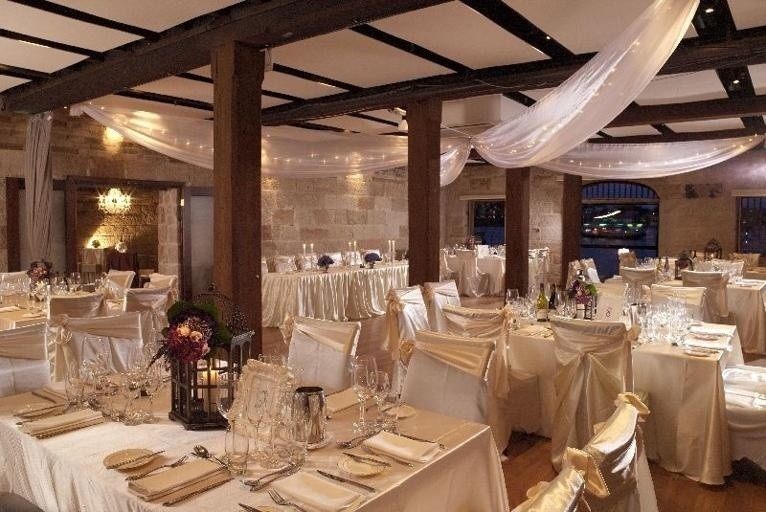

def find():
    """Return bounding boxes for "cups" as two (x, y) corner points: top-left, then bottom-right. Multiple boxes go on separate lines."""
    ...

(505, 288), (579, 329)
(623, 287), (690, 344)
(67, 342), (165, 426)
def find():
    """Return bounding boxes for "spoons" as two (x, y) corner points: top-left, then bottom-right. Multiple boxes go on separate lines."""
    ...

(360, 442), (414, 467)
(124, 455), (186, 480)
(193, 444), (226, 466)
(242, 466), (296, 487)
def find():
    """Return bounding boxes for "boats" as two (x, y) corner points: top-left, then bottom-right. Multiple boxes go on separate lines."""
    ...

(582, 220), (647, 238)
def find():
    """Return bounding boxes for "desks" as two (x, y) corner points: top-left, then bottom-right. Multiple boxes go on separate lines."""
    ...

(0, 357), (509, 511)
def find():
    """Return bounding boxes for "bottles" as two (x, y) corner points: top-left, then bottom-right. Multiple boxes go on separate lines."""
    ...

(548, 283), (561, 322)
(537, 284), (548, 322)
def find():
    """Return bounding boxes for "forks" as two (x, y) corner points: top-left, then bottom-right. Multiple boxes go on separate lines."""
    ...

(267, 487), (306, 512)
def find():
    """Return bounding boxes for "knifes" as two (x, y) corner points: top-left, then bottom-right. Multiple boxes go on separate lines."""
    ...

(392, 430), (446, 448)
(317, 468), (375, 493)
(161, 477), (234, 506)
(250, 464), (303, 491)
(105, 449), (165, 470)
(30, 392), (57, 404)
(239, 502), (265, 512)
(13, 403), (65, 417)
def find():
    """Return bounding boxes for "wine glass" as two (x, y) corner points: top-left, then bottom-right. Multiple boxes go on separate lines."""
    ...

(0, 273), (109, 320)
(214, 354), (402, 474)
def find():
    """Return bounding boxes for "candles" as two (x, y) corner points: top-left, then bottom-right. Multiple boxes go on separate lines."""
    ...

(392, 239), (396, 262)
(310, 242), (314, 256)
(388, 239), (391, 262)
(302, 243), (306, 256)
(347, 241), (352, 256)
(353, 241), (357, 255)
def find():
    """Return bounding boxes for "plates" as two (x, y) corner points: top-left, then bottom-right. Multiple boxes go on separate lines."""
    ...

(695, 334), (722, 341)
(686, 347), (712, 356)
(103, 449), (155, 469)
(16, 401), (56, 417)
(388, 405), (416, 419)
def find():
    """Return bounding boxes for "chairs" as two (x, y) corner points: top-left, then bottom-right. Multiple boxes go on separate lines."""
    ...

(2, 245), (507, 392)
(402, 246), (766, 511)
